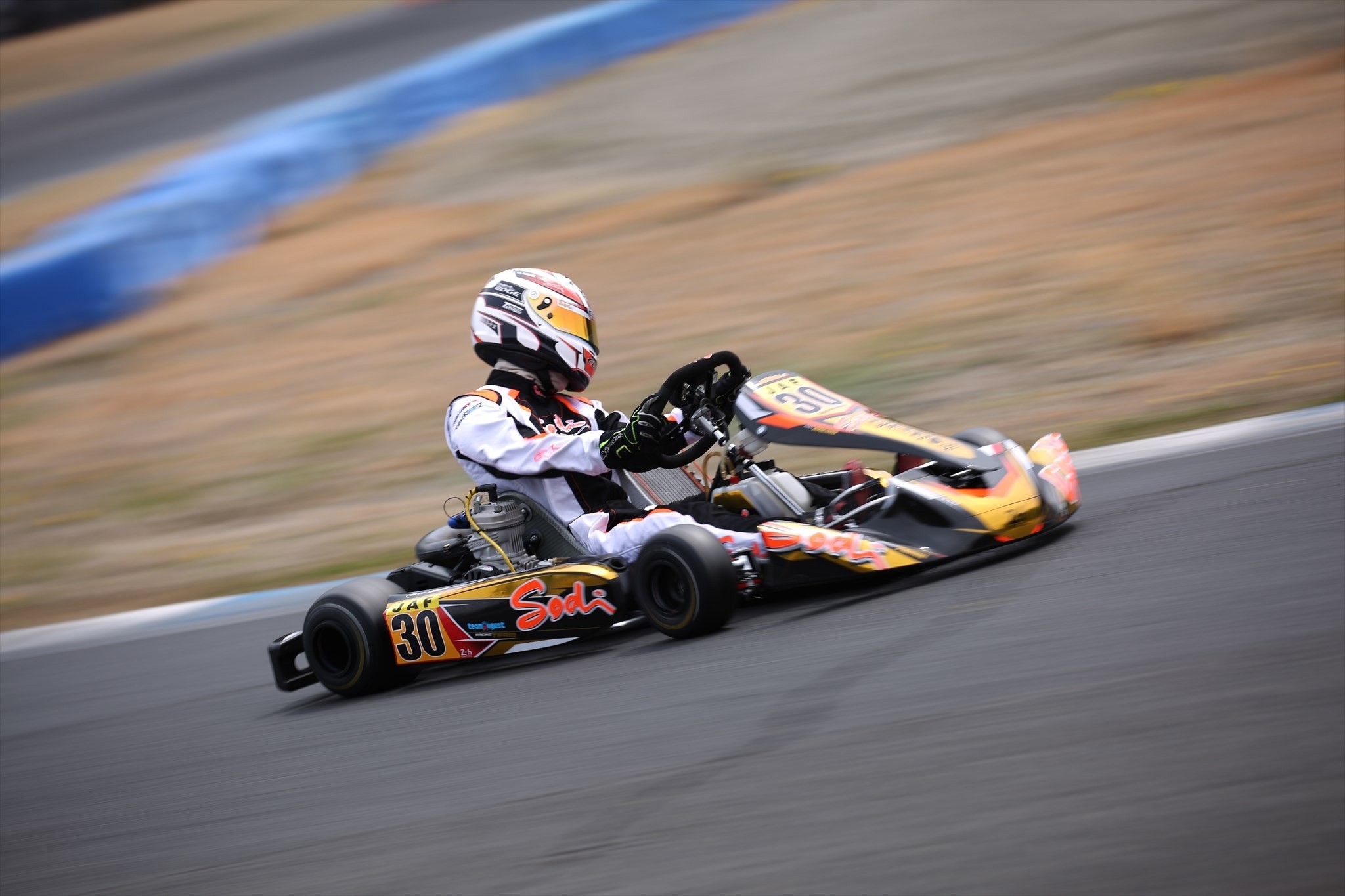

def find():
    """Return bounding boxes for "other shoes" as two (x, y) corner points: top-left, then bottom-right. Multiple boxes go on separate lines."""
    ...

(829, 459), (868, 526)
(890, 451), (923, 478)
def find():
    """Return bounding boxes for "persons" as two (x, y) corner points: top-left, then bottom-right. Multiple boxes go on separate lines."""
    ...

(444, 268), (923, 571)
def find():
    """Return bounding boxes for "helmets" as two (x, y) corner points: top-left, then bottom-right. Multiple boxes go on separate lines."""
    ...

(471, 267), (601, 392)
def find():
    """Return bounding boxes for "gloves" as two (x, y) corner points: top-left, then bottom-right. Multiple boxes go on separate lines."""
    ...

(709, 363), (752, 414)
(598, 391), (672, 471)
